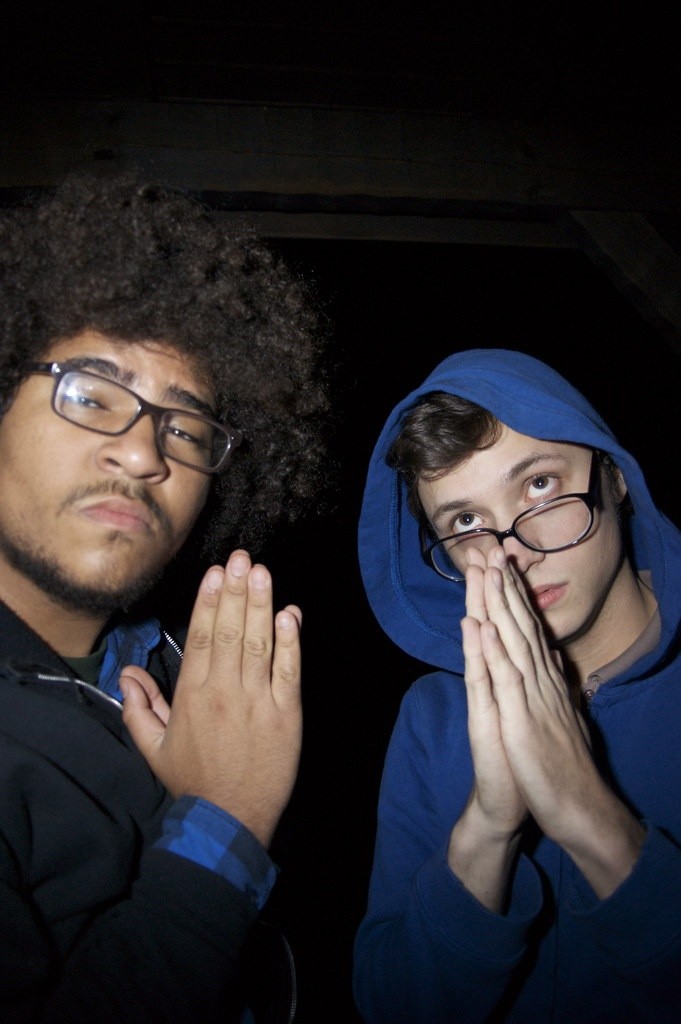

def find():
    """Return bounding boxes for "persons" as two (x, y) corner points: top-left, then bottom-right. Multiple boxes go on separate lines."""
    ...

(352, 348), (681, 1024)
(0, 170), (331, 1024)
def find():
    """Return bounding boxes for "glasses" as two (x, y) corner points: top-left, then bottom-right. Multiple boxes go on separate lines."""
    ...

(17, 361), (241, 474)
(418, 448), (600, 584)
